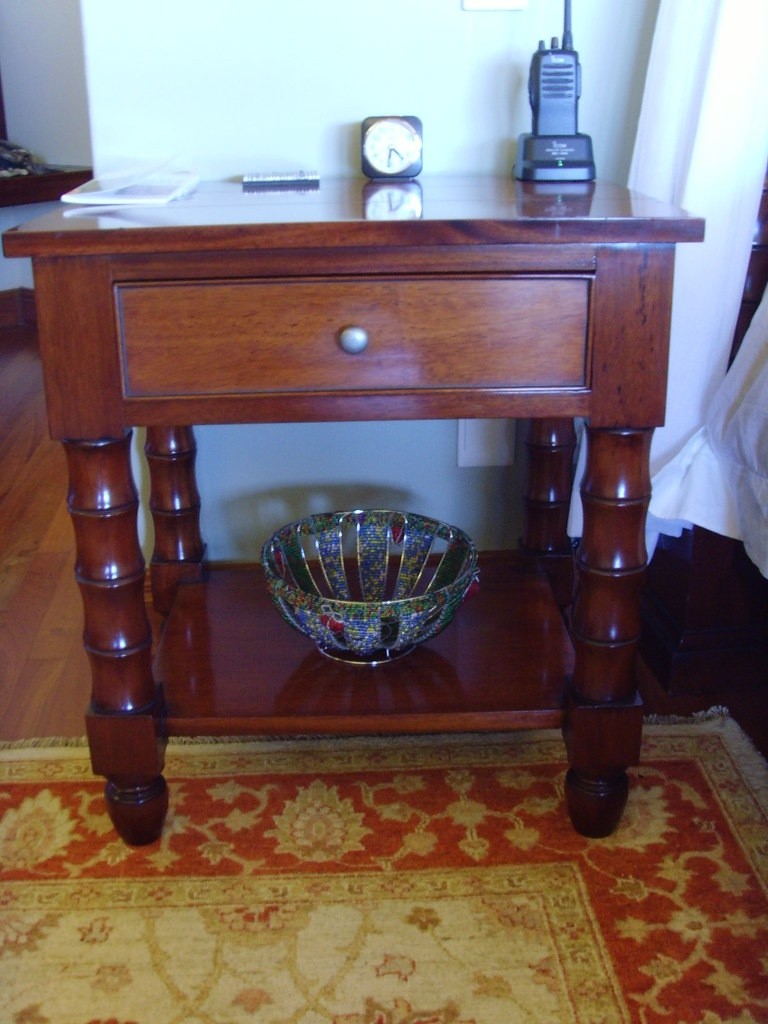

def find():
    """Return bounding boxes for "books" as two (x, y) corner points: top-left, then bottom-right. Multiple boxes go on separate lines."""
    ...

(61, 175), (200, 204)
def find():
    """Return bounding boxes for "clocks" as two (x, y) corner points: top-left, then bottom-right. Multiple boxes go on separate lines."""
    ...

(361, 114), (429, 181)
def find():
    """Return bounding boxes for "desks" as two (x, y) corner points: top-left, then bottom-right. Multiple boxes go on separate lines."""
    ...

(0, 178), (707, 843)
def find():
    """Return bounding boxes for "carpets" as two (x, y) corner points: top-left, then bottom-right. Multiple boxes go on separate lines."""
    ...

(0, 704), (767, 1023)
